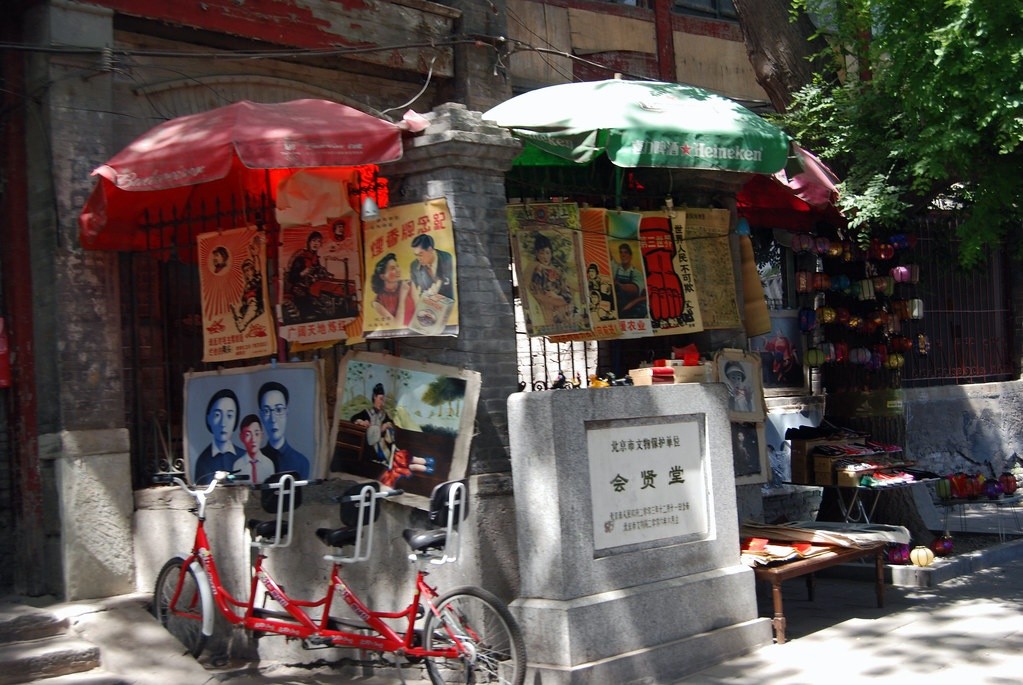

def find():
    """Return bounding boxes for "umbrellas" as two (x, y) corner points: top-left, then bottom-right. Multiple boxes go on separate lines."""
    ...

(78, 98), (430, 263)
(736, 147), (841, 229)
(482, 72), (790, 206)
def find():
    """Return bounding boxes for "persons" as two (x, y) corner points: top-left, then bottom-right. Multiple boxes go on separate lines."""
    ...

(724, 361), (753, 412)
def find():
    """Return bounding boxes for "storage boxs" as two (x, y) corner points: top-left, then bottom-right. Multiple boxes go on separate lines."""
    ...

(794, 437), (892, 487)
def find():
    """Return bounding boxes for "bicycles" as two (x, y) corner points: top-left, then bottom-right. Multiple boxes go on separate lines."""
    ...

(152, 470), (527, 685)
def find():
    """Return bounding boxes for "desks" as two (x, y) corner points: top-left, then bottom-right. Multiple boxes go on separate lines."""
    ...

(780, 478), (942, 556)
(739, 539), (888, 644)
(933, 496), (1023, 545)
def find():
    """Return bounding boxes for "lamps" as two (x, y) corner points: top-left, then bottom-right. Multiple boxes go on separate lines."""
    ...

(360, 199), (379, 221)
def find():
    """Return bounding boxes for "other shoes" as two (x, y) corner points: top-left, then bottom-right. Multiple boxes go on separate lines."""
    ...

(785, 419), (872, 441)
(834, 457), (916, 471)
(860, 467), (941, 490)
(813, 440), (904, 457)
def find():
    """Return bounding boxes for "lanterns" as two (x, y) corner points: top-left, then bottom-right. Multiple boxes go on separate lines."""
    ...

(889, 537), (952, 566)
(936, 472), (1017, 500)
(793, 232), (931, 373)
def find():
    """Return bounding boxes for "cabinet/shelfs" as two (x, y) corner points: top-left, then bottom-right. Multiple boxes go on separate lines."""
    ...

(813, 446), (915, 470)
